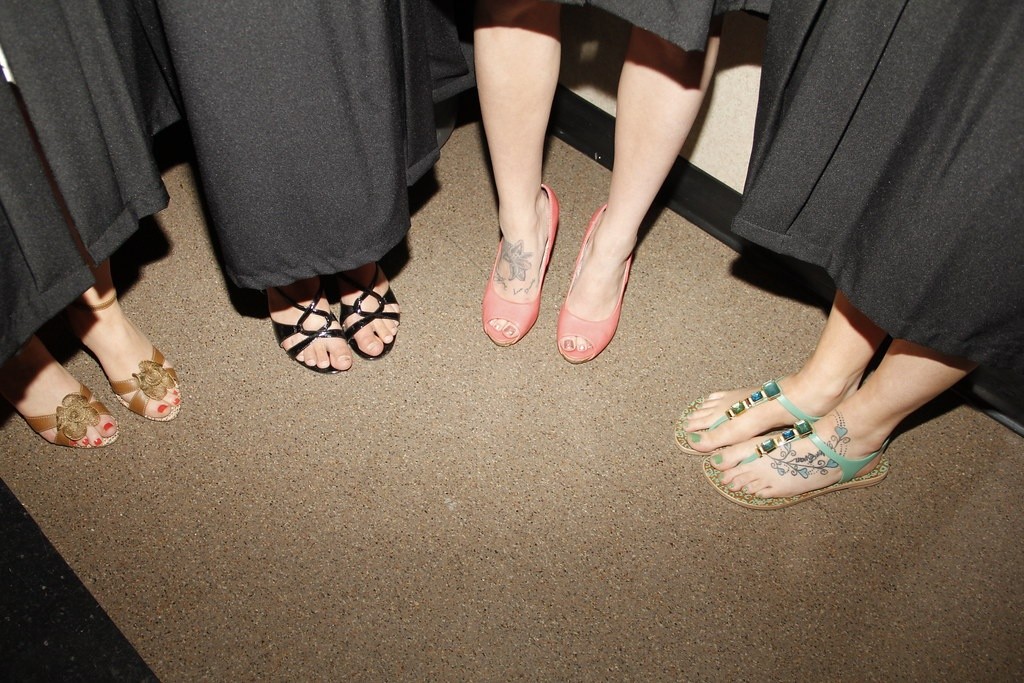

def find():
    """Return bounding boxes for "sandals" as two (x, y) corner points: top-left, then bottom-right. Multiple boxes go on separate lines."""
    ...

(674, 375), (820, 456)
(61, 288), (181, 422)
(332, 262), (401, 360)
(263, 275), (353, 375)
(703, 419), (890, 510)
(0, 334), (120, 449)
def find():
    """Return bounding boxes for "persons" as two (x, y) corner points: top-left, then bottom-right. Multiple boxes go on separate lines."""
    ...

(469, 0), (768, 364)
(135, 0), (476, 376)
(676, 0), (1024, 509)
(0, 0), (183, 450)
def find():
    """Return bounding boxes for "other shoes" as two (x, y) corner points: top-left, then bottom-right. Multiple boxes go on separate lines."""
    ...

(482, 185), (558, 347)
(557, 204), (632, 364)
